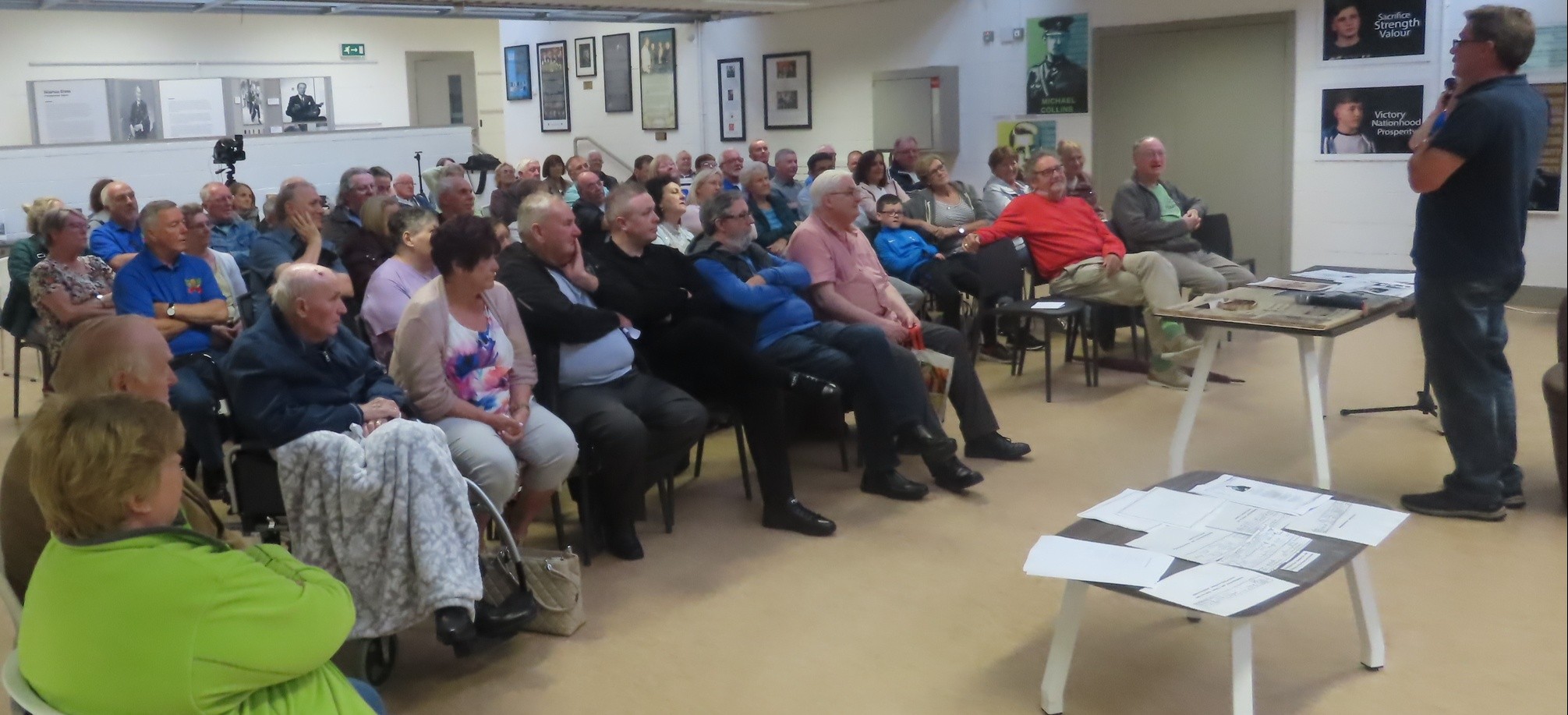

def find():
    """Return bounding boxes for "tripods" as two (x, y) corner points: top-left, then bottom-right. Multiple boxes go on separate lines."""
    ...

(1341, 368), (1437, 420)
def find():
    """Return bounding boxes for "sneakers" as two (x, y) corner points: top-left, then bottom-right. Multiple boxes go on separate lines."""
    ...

(1444, 464), (1526, 510)
(1165, 339), (1205, 365)
(979, 339), (1017, 363)
(1145, 366), (1209, 392)
(1401, 485), (1507, 522)
(1005, 331), (1046, 351)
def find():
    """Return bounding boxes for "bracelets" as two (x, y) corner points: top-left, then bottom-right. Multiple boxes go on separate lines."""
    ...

(509, 403), (530, 415)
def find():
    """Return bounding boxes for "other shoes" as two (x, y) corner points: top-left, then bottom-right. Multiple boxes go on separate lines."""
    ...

(607, 521), (643, 560)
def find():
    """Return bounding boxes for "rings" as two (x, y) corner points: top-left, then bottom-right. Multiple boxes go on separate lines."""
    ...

(964, 244), (967, 247)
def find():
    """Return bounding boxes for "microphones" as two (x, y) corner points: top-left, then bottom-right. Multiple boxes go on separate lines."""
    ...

(1296, 292), (1365, 310)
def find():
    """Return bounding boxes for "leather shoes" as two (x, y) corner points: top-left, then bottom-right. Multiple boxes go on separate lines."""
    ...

(901, 424), (957, 460)
(786, 370), (842, 407)
(965, 431), (1031, 459)
(435, 606), (476, 646)
(762, 494), (836, 537)
(861, 467), (928, 500)
(474, 600), (537, 637)
(934, 457), (984, 491)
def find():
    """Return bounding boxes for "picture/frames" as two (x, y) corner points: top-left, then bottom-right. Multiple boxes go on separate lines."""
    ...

(575, 37), (597, 77)
(602, 33), (633, 113)
(505, 45), (533, 100)
(639, 28), (678, 130)
(762, 50), (812, 130)
(536, 40), (571, 132)
(717, 58), (745, 142)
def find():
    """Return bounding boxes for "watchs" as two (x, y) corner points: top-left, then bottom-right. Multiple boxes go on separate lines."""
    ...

(958, 225), (966, 236)
(166, 302), (175, 319)
(95, 294), (108, 308)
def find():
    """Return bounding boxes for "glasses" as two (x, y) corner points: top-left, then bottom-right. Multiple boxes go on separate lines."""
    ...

(66, 222), (90, 232)
(928, 164), (945, 177)
(1452, 39), (1489, 48)
(1035, 164), (1065, 177)
(830, 189), (862, 196)
(703, 162), (718, 167)
(722, 157), (744, 164)
(723, 209), (753, 219)
(880, 210), (903, 216)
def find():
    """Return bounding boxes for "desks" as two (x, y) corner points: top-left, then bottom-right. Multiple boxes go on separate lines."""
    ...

(1158, 267), (1422, 488)
(1040, 471), (1391, 715)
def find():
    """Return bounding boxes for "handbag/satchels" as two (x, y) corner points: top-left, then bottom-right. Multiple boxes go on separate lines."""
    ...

(906, 325), (955, 424)
(959, 300), (982, 368)
(482, 546), (586, 637)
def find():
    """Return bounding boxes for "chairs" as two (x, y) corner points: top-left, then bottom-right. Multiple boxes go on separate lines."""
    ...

(0, 212), (1255, 715)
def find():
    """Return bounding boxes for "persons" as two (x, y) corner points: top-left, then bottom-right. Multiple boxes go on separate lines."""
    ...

(1321, 3), (1371, 60)
(1319, 90), (1382, 153)
(1400, 5), (1550, 522)
(0, 138), (1264, 715)
